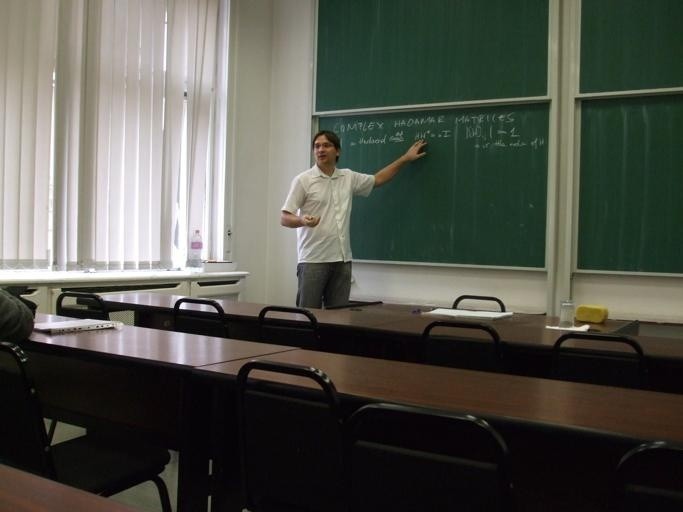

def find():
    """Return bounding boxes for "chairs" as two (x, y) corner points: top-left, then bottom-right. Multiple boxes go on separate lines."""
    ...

(0, 292), (683, 512)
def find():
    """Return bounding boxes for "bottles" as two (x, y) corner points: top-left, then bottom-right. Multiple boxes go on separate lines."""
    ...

(189, 228), (203, 269)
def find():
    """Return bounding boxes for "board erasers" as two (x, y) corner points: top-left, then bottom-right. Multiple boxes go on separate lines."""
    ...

(575, 304), (609, 324)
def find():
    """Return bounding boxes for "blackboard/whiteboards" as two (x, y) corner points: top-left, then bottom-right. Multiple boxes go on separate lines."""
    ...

(317, 101), (552, 270)
(579, 0), (683, 97)
(573, 90), (683, 276)
(315, 0), (553, 112)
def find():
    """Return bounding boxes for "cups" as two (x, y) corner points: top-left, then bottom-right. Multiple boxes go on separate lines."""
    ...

(559, 297), (575, 329)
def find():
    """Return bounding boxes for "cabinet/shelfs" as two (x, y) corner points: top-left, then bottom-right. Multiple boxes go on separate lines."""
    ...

(0, 270), (250, 317)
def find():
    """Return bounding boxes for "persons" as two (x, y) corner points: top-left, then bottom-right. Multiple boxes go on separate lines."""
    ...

(279, 131), (428, 309)
(0, 288), (34, 345)
